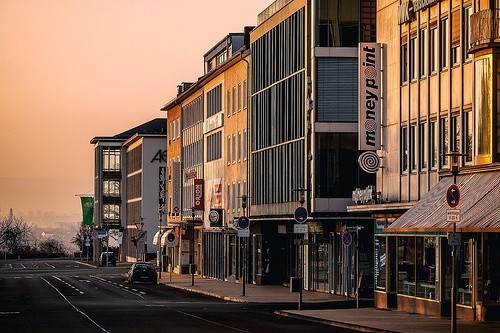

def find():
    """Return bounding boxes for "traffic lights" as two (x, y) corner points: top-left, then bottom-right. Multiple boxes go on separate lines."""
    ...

(131, 236), (137, 247)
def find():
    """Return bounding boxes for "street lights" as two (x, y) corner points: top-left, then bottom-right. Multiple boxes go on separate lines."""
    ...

(236, 194), (253, 297)
(102, 227), (111, 267)
(187, 207), (199, 286)
(137, 217), (148, 262)
(155, 211), (168, 279)
(292, 188), (312, 310)
(440, 152), (469, 333)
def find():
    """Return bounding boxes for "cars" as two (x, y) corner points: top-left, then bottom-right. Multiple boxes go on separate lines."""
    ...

(127, 262), (158, 284)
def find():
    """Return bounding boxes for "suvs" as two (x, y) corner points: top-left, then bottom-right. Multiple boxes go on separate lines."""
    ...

(98, 252), (117, 267)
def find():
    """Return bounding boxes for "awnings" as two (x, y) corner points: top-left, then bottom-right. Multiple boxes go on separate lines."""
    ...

(153, 230), (164, 245)
(161, 229), (174, 247)
(383, 171), (500, 232)
